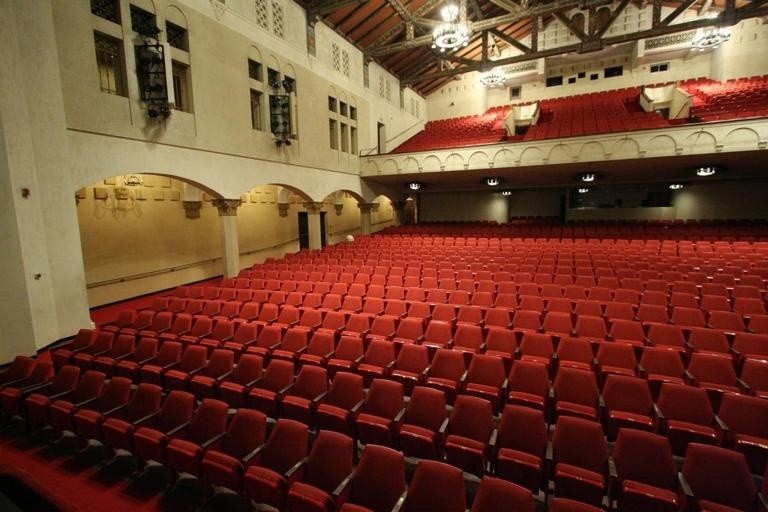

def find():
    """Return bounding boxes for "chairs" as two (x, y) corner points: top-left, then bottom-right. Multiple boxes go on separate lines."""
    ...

(384, 76), (768, 156)
(1, 214), (767, 511)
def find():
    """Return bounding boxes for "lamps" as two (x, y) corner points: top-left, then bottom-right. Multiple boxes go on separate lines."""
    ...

(502, 190), (512, 195)
(669, 184), (684, 190)
(487, 177), (500, 186)
(697, 165), (716, 177)
(480, 66), (509, 87)
(430, 2), (471, 54)
(410, 182), (421, 189)
(406, 197), (413, 201)
(690, 8), (731, 52)
(582, 173), (595, 182)
(578, 188), (588, 194)
